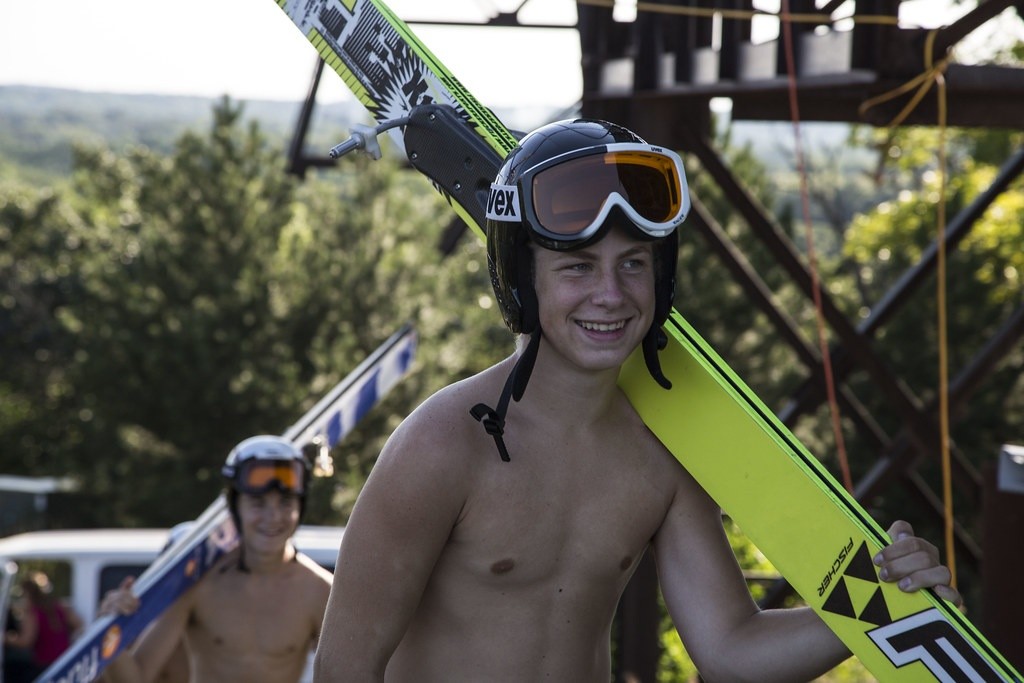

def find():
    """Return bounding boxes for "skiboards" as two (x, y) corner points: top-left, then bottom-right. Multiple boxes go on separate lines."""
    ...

(274, 0), (1024, 683)
(31, 325), (421, 683)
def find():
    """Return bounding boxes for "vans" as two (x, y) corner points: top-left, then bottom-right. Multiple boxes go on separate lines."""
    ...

(0, 524), (345, 683)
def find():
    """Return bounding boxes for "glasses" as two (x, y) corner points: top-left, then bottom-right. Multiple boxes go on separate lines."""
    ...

(235, 457), (307, 495)
(516, 142), (692, 250)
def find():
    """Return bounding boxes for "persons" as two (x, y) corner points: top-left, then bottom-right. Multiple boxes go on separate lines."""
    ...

(96, 434), (333, 683)
(0, 580), (85, 683)
(312, 117), (963, 683)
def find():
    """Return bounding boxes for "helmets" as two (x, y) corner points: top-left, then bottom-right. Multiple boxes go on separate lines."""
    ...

(486, 119), (680, 335)
(218, 434), (309, 532)
